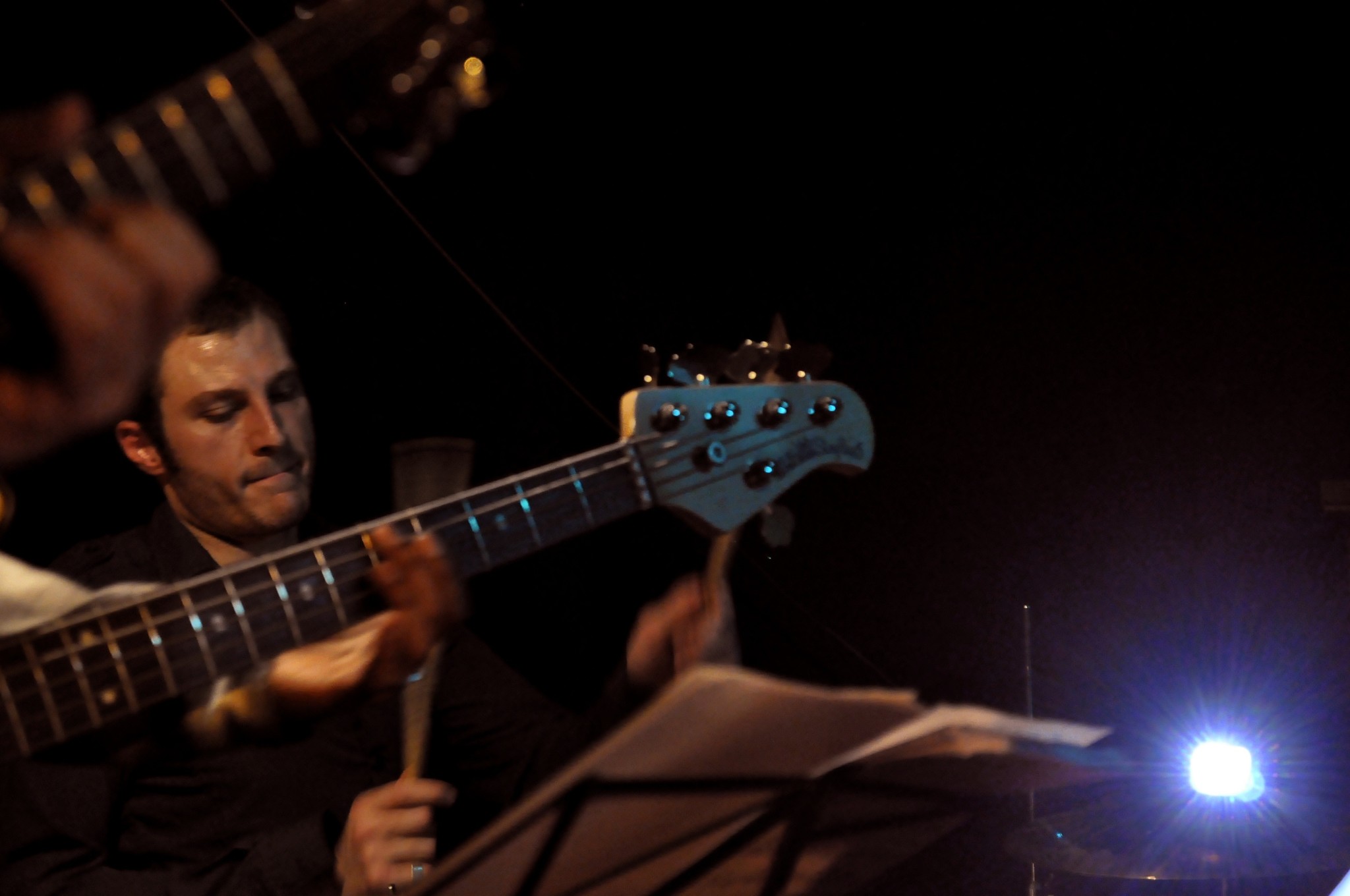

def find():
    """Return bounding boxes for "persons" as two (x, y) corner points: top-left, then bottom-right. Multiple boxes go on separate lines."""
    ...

(1, 85), (219, 469)
(0, 522), (464, 771)
(0, 271), (748, 896)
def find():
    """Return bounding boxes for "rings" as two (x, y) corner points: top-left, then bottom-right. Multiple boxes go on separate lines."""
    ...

(409, 860), (424, 886)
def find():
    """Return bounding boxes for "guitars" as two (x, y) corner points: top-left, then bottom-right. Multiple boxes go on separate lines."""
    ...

(3, 340), (876, 753)
(0, 0), (488, 299)
